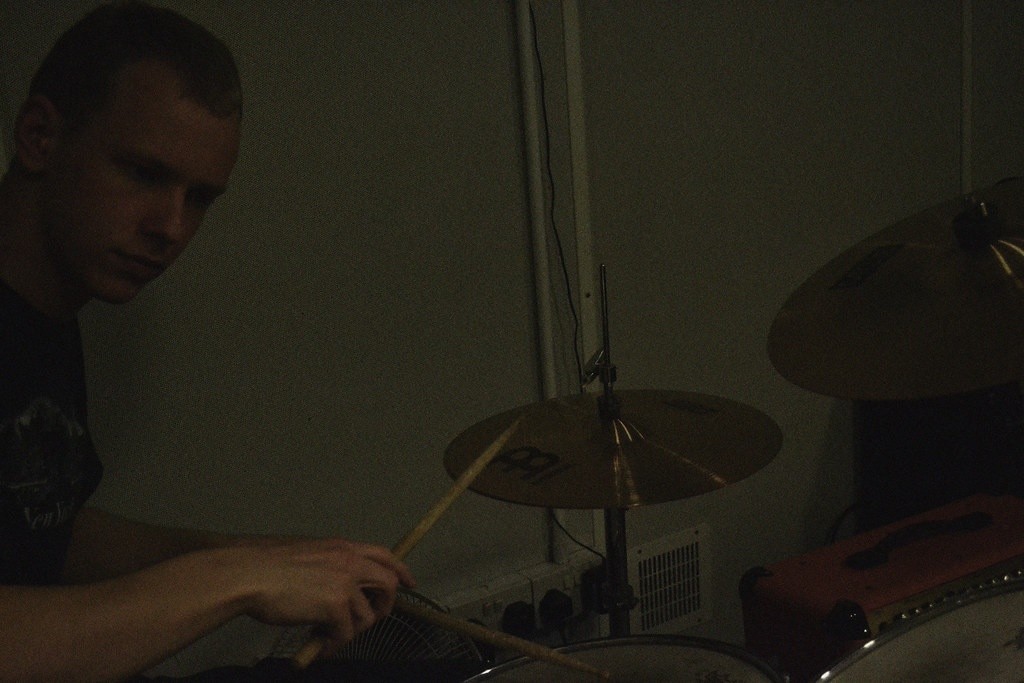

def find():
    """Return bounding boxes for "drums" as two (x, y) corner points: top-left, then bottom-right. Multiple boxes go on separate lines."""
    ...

(443, 562), (1024, 683)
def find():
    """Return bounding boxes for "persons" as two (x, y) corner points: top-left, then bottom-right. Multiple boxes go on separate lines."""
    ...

(0, 1), (420, 683)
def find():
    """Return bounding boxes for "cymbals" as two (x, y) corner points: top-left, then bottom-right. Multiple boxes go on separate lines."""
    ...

(764, 173), (1024, 402)
(439, 387), (784, 511)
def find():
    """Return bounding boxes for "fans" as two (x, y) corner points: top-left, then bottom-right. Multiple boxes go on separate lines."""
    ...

(263, 581), (487, 664)
